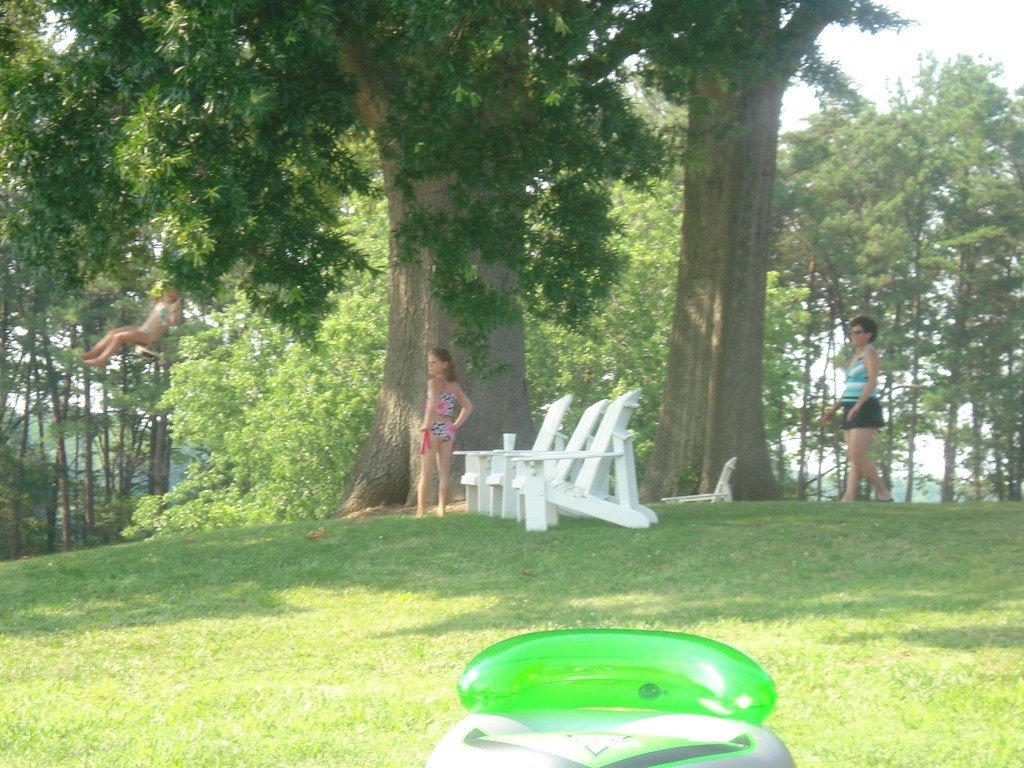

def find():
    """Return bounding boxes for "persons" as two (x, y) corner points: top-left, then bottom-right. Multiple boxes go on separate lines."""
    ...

(76, 279), (184, 367)
(414, 349), (474, 518)
(819, 315), (894, 502)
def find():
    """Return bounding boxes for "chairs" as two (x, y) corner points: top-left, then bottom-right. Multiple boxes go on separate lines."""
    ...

(454, 387), (659, 531)
(660, 456), (737, 505)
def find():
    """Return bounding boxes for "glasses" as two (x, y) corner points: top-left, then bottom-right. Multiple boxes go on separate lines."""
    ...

(849, 330), (865, 335)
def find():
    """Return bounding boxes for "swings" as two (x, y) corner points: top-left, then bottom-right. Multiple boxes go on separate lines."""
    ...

(132, 286), (188, 359)
